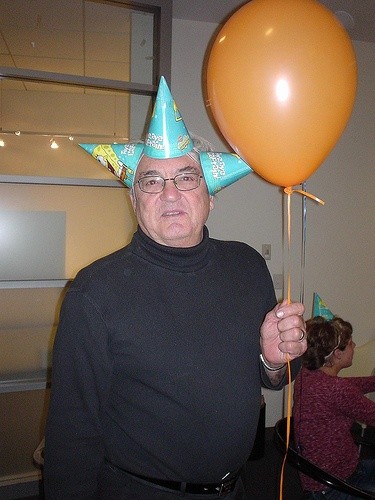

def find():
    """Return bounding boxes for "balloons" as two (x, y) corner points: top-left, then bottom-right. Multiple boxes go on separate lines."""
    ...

(207, 0), (357, 194)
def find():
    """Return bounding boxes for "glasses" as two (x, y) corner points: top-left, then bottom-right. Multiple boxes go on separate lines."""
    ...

(134, 173), (203, 194)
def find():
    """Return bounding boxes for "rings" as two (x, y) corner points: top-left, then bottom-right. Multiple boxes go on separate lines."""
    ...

(299, 330), (305, 341)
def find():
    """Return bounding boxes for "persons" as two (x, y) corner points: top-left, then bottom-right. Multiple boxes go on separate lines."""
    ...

(41, 76), (308, 499)
(294, 293), (375, 500)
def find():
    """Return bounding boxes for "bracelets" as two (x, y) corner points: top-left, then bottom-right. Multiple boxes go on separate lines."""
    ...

(260, 354), (286, 372)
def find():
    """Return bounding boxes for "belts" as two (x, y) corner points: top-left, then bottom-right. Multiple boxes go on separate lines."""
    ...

(115, 465), (242, 495)
